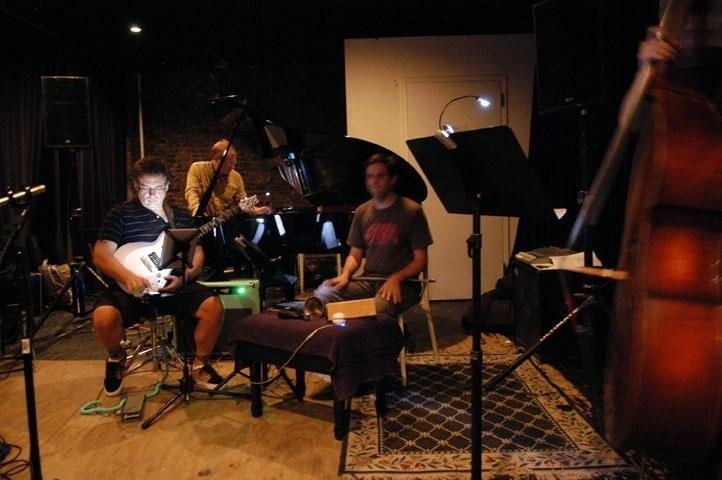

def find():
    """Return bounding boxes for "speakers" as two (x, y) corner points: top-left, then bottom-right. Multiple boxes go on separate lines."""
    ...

(41, 76), (92, 149)
(531, 0), (630, 115)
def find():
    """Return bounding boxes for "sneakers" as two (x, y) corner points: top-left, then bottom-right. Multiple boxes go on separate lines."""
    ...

(103, 348), (127, 396)
(189, 364), (225, 390)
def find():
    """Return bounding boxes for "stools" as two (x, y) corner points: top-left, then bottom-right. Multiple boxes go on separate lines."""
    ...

(229, 300), (407, 441)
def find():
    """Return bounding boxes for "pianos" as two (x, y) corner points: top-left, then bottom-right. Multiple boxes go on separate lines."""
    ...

(191, 94), (427, 301)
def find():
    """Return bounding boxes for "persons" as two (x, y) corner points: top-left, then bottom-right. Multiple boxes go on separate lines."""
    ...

(183, 137), (275, 274)
(610, 0), (720, 480)
(90, 154), (228, 398)
(309, 151), (434, 409)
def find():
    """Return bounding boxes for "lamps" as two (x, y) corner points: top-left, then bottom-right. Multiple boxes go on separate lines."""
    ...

(437, 94), (491, 137)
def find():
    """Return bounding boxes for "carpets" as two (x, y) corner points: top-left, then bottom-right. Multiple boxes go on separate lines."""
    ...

(339, 352), (641, 479)
(20, 311), (172, 361)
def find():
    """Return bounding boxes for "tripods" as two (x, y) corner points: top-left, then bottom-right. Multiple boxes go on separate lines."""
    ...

(30, 152), (111, 348)
(140, 243), (252, 429)
(485, 112), (611, 434)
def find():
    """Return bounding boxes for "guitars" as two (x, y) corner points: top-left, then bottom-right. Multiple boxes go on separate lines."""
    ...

(112, 195), (259, 298)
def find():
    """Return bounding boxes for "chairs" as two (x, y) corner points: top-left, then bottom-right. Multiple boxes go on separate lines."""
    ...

(83, 226), (185, 370)
